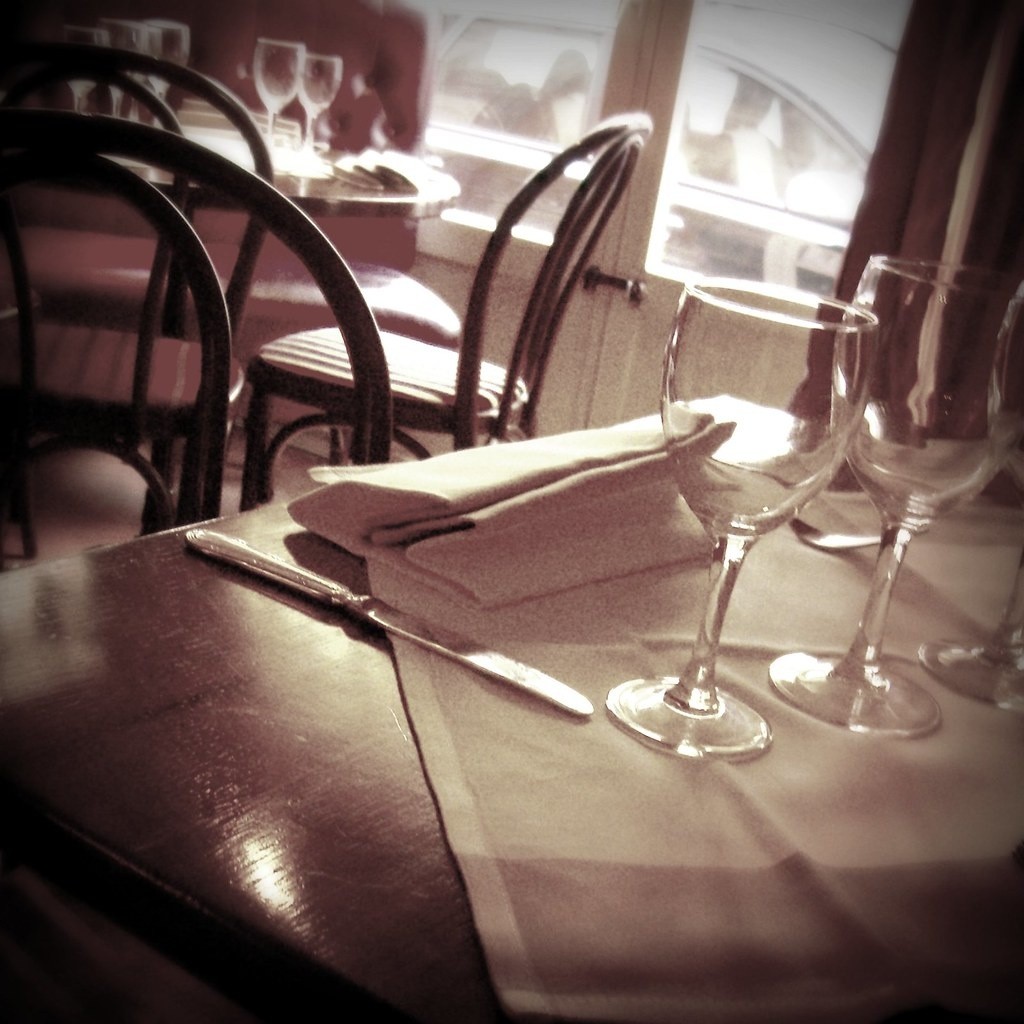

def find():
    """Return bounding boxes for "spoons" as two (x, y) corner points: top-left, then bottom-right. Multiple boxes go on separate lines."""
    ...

(605, 275), (880, 759)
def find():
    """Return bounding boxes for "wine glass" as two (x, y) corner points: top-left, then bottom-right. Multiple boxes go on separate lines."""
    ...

(296, 48), (343, 170)
(56, 23), (113, 120)
(769, 249), (1024, 739)
(918, 279), (1024, 713)
(100, 17), (160, 122)
(140, 18), (190, 129)
(252, 36), (305, 167)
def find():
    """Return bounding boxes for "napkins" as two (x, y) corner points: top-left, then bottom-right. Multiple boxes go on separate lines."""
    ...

(289, 146), (734, 619)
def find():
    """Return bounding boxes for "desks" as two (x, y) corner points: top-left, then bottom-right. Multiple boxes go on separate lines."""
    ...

(0, 395), (1024, 1024)
(100, 109), (464, 524)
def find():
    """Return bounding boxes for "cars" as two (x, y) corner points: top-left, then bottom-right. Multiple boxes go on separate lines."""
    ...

(417, 2), (900, 304)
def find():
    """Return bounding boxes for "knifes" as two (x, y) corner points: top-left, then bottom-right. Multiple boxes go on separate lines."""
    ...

(185, 529), (599, 718)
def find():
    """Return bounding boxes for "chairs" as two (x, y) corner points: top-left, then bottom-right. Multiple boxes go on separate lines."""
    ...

(0, 44), (654, 582)
